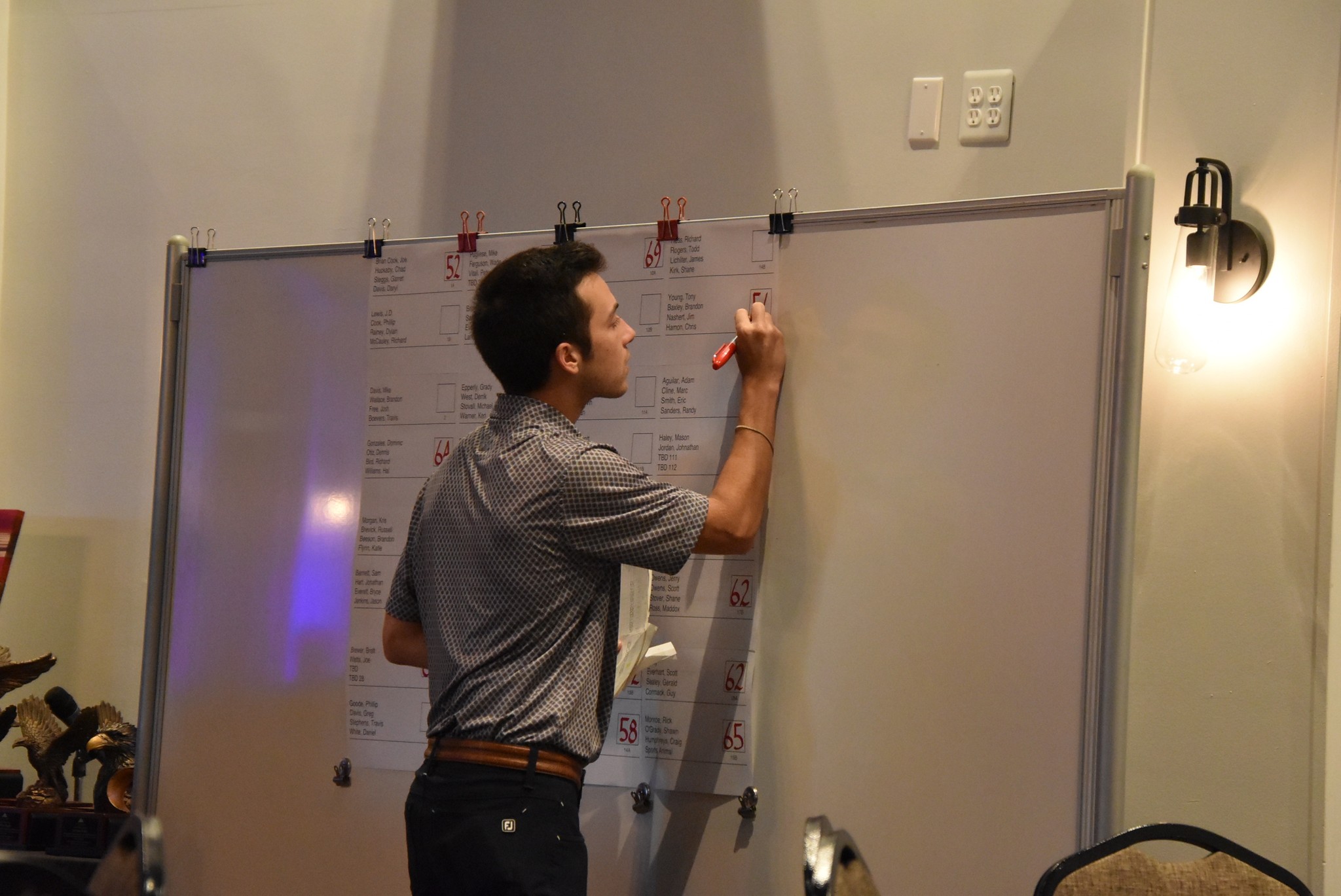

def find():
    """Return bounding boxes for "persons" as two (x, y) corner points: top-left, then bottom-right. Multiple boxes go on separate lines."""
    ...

(382, 240), (785, 896)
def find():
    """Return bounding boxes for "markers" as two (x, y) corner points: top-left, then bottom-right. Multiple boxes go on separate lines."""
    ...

(711, 336), (738, 370)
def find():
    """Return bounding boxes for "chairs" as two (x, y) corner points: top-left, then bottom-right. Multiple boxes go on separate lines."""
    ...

(801, 811), (1314, 896)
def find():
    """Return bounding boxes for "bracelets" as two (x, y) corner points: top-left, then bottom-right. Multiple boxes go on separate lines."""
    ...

(735, 425), (774, 455)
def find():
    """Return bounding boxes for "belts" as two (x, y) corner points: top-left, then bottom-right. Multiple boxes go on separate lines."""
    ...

(424, 738), (584, 788)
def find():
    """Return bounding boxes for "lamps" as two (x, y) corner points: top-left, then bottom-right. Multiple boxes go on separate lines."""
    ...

(1155, 158), (1269, 374)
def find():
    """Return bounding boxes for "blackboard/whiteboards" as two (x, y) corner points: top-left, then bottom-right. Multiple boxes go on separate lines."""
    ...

(131, 167), (1154, 896)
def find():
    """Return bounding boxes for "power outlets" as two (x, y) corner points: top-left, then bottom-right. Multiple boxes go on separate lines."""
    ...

(958, 67), (1014, 142)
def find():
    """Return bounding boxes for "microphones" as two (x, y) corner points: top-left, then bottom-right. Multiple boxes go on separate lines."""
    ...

(44, 687), (81, 726)
